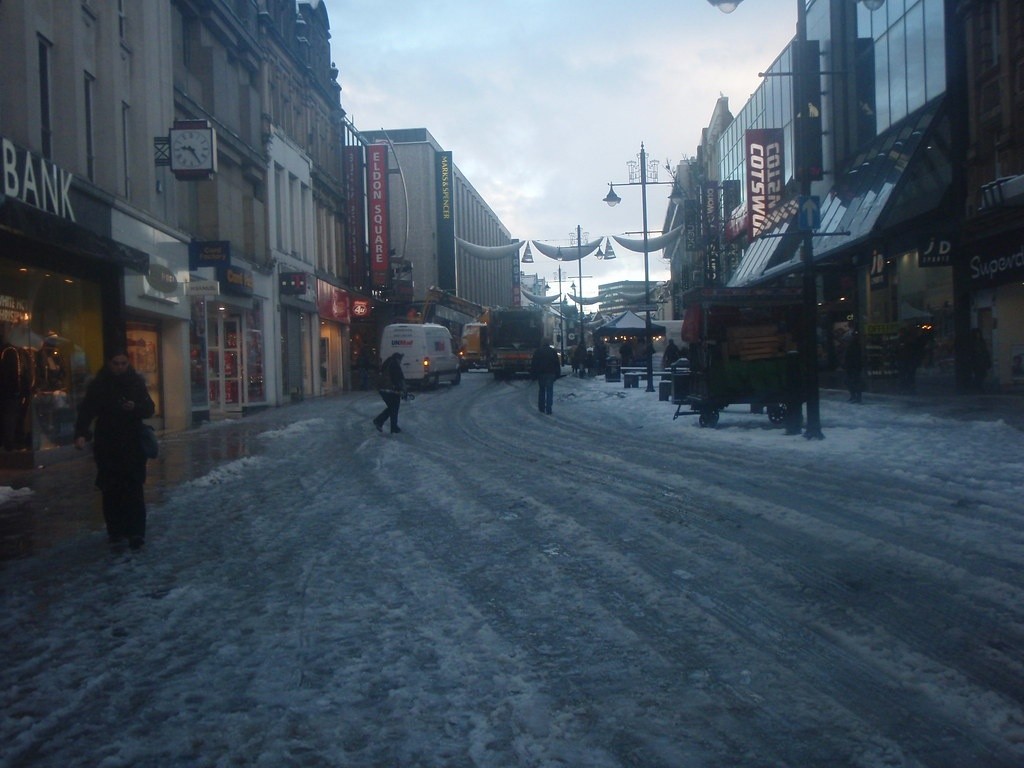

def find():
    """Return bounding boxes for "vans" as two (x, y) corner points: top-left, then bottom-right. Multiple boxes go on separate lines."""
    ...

(462, 320), (491, 372)
(380, 322), (459, 392)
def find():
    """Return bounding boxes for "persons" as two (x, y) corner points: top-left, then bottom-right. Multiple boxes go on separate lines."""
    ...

(570, 337), (690, 382)
(842, 330), (862, 401)
(35, 329), (68, 448)
(968, 327), (991, 395)
(1012, 355), (1024, 377)
(529, 337), (561, 415)
(74, 343), (155, 551)
(373, 352), (407, 433)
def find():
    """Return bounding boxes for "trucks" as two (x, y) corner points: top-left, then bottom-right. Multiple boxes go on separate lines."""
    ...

(487, 304), (554, 379)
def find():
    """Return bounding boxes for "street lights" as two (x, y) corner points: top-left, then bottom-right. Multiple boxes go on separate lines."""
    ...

(553, 225), (604, 374)
(607, 140), (685, 393)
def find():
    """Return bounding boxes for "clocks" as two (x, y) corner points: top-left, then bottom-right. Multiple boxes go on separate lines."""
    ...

(167, 119), (218, 182)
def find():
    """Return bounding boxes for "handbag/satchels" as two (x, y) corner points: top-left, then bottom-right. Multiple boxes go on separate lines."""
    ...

(142, 426), (158, 461)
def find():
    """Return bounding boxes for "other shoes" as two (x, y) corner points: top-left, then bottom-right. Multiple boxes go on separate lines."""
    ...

(113, 534), (145, 552)
(374, 421), (403, 433)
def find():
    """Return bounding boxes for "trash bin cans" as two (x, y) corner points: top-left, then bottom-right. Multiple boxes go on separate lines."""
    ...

(669, 357), (693, 406)
(605, 355), (621, 383)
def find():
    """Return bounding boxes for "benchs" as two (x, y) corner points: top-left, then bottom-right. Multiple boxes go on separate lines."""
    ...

(620, 363), (693, 405)
